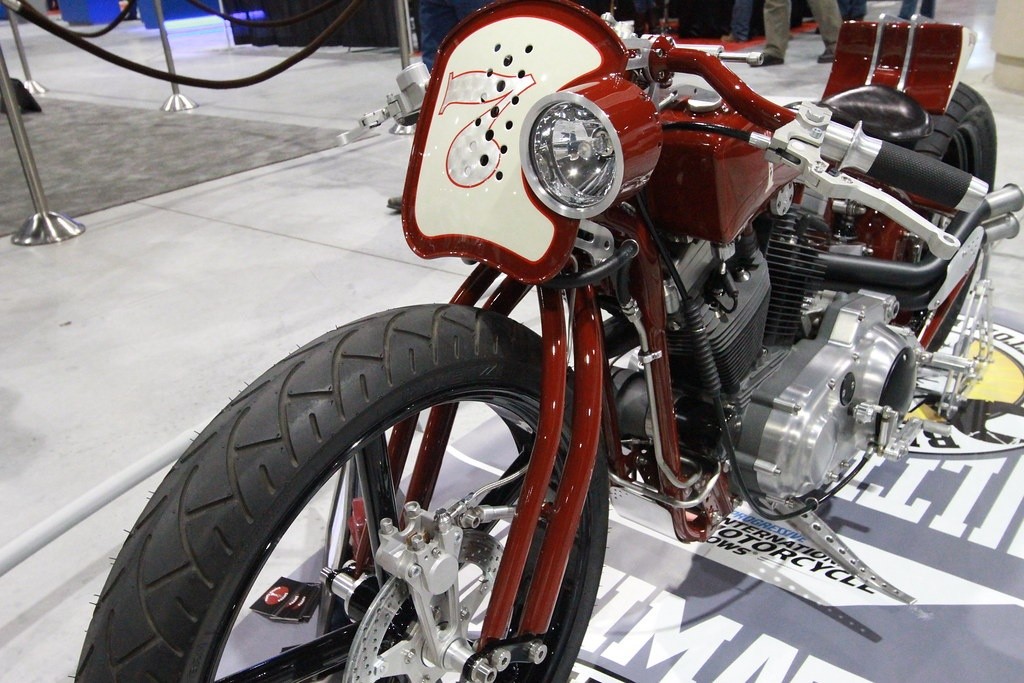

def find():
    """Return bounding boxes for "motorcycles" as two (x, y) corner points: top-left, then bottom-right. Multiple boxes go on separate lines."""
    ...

(75, 1), (1024, 683)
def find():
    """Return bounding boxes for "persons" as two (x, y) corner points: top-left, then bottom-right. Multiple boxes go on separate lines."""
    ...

(751, 0), (843, 68)
(721, 0), (794, 42)
(814, 0), (867, 33)
(899, 0), (937, 20)
(419, 0), (494, 72)
(125, 0), (137, 20)
(633, 0), (658, 37)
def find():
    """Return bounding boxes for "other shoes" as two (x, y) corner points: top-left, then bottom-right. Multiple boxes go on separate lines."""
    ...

(818, 49), (836, 62)
(747, 53), (784, 66)
(720, 34), (738, 43)
